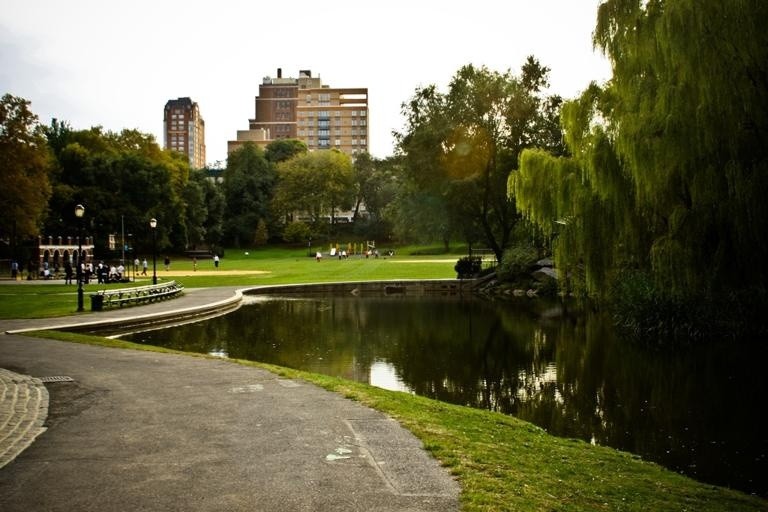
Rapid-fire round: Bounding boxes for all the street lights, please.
[75,203,85,312]
[149,217,157,284]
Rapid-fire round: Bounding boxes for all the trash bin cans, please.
[89,295,104,312]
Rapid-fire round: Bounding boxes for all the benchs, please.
[99,280,184,310]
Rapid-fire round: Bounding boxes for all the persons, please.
[213,253,219,267]
[164,255,170,271]
[10,260,19,280]
[134,255,139,275]
[140,255,148,275]
[41,257,125,286]
[314,244,384,263]
